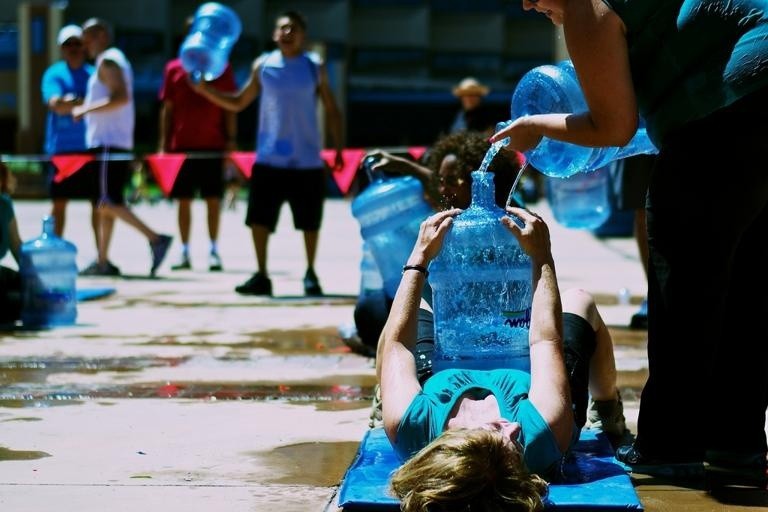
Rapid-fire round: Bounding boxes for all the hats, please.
[57,25,83,46]
[453,78,488,97]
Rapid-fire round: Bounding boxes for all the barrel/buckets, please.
[179,4,243,86]
[547,171,611,232]
[428,171,539,369]
[496,61,657,179]
[360,245,384,311]
[351,155,437,300]
[20,217,79,328]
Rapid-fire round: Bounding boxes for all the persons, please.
[620,155,648,329]
[157,15,238,271]
[449,78,492,133]
[0,162,49,337]
[373,204,633,511]
[189,8,346,300]
[70,15,174,276]
[41,23,97,274]
[356,130,521,209]
[492,0,768,491]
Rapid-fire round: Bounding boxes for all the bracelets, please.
[400,264,430,279]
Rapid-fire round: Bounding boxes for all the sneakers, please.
[585,387,625,438]
[80,260,118,276]
[616,445,647,465]
[150,236,173,272]
[338,322,376,357]
[172,254,221,270]
[304,277,321,295]
[236,272,271,294]
[370,384,382,427]
